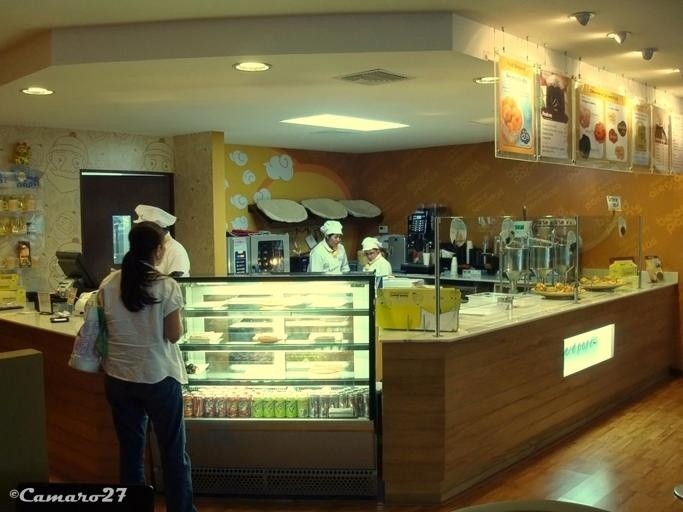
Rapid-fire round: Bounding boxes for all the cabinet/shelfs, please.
[0,171,44,271]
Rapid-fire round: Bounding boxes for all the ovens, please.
[226,230,290,274]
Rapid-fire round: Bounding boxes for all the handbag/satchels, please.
[69,305,102,374]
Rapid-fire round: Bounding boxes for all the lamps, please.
[569,11,656,61]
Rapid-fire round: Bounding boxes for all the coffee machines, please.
[405,205,450,271]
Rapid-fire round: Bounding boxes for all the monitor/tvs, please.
[55,250,99,290]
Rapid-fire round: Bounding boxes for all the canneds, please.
[183,387,369,419]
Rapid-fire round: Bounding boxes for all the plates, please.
[578,276,623,290]
[529,285,587,299]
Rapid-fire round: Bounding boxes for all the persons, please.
[133,204,192,278]
[96,220,199,512]
[362,236,393,276]
[306,220,351,273]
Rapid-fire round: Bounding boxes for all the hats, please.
[132,205,177,228]
[320,220,344,237]
[361,237,379,251]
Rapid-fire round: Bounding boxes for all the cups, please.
[450,257,458,276]
[422,253,430,266]
[499,96,524,145]
[466,240,474,265]
